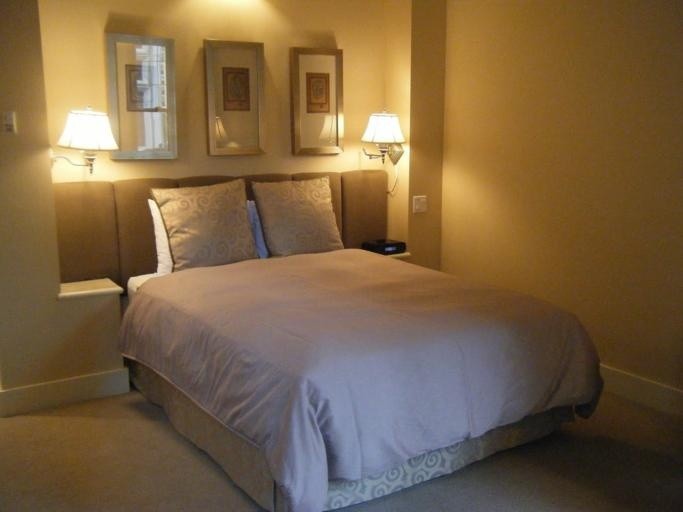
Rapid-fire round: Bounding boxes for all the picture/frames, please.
[106,31,178,161]
[203,39,267,156]
[289,45,345,156]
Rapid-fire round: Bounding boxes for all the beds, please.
[53,169,603,512]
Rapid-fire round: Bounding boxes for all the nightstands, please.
[389,251,413,259]
[58,277,131,404]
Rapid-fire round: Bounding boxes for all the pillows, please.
[146,176,345,273]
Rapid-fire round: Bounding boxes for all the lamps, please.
[361,110,406,165]
[50,107,119,174]
